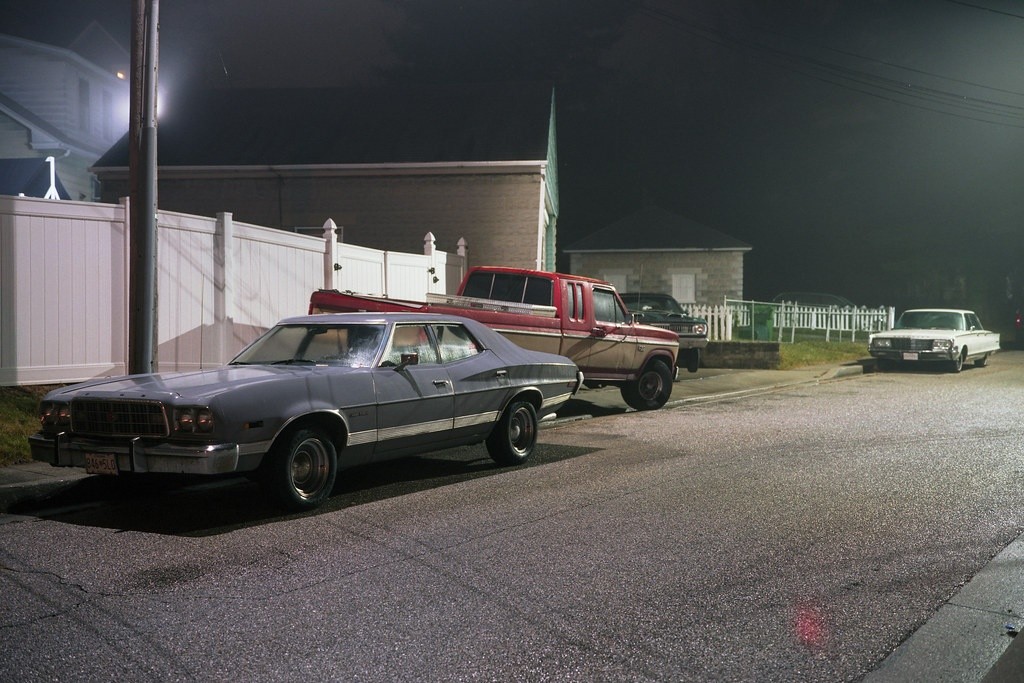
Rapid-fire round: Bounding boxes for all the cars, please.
[867,308,1000,374]
[619,292,709,373]
[28,311,586,515]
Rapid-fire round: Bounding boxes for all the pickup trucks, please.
[307,264,682,411]
